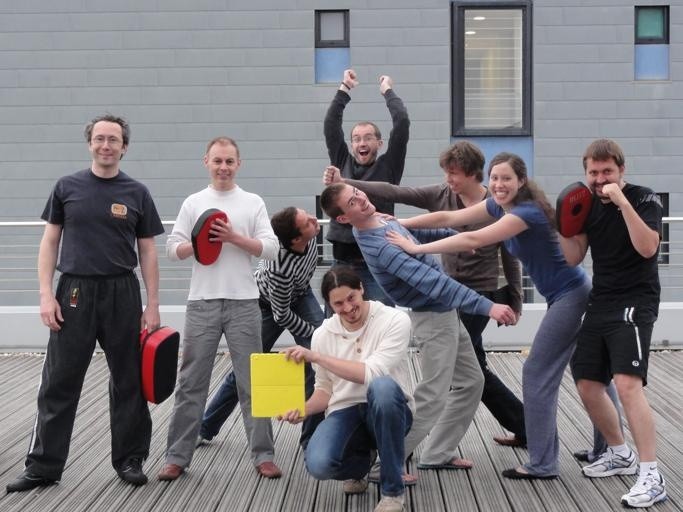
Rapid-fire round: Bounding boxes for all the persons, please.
[557,139,668,509]
[191,206,326,450]
[4,115,165,493]
[156,137,283,481]
[273,264,416,512]
[371,153,623,481]
[321,140,527,448]
[321,68,411,318]
[315,181,518,486]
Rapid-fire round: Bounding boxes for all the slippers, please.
[417,457,472,470]
[367,472,418,485]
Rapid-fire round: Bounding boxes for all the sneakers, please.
[502,467,557,480]
[582,448,637,477]
[343,473,368,493]
[373,493,405,512]
[574,448,595,463]
[117,460,148,485]
[620,473,667,507]
[6,470,48,492]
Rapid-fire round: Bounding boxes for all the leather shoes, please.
[256,462,282,478]
[492,434,518,446]
[158,462,181,479]
